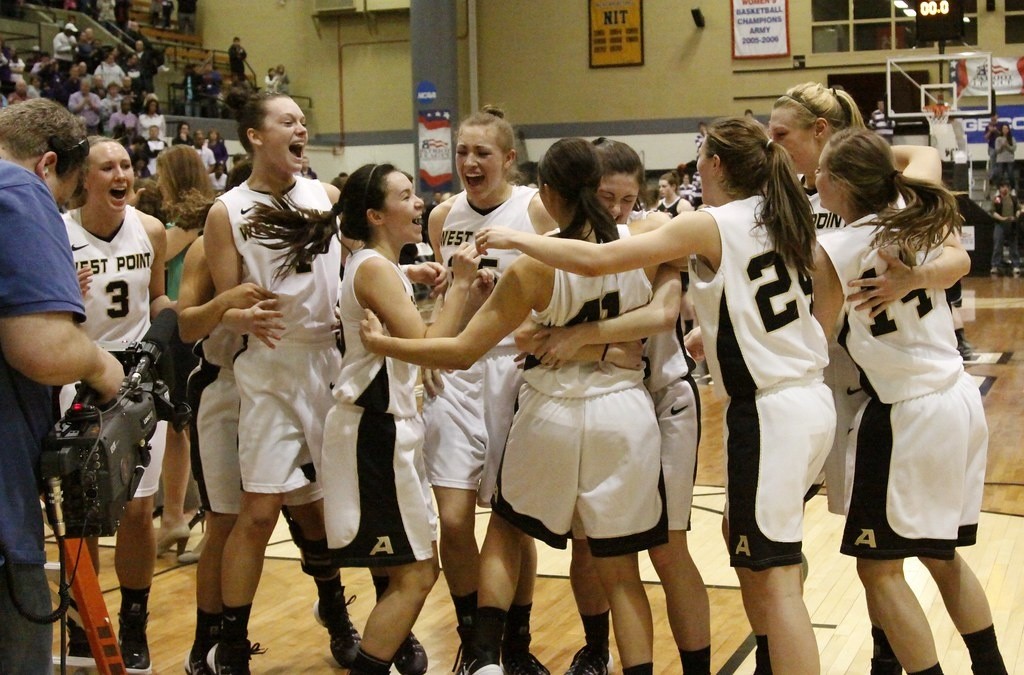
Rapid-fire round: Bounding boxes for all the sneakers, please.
[207,638,268,675]
[990,267,998,273]
[184,651,213,675]
[565,636,610,675]
[870,656,902,675]
[454,631,503,675]
[1012,267,1020,273]
[117,611,152,675]
[501,634,550,675]
[1010,188,1016,196]
[314,586,361,669]
[67,614,92,658]
[392,630,427,675]
[994,190,999,196]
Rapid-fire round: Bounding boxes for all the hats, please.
[66,23,77,32]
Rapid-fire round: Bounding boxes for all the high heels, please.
[155,525,190,556]
[152,505,163,523]
[187,506,206,533]
[177,551,201,562]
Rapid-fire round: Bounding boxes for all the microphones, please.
[130,307,179,390]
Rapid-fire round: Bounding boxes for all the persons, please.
[1,0,452,557]
[58,134,177,675]
[0,97,125,675]
[421,104,560,675]
[320,165,484,675]
[360,136,670,675]
[868,99,1024,278]
[204,92,428,675]
[632,109,802,382]
[682,83,972,675]
[474,118,838,675]
[178,155,364,674]
[810,128,1010,675]
[514,138,711,675]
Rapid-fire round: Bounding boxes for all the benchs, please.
[97,0,258,94]
